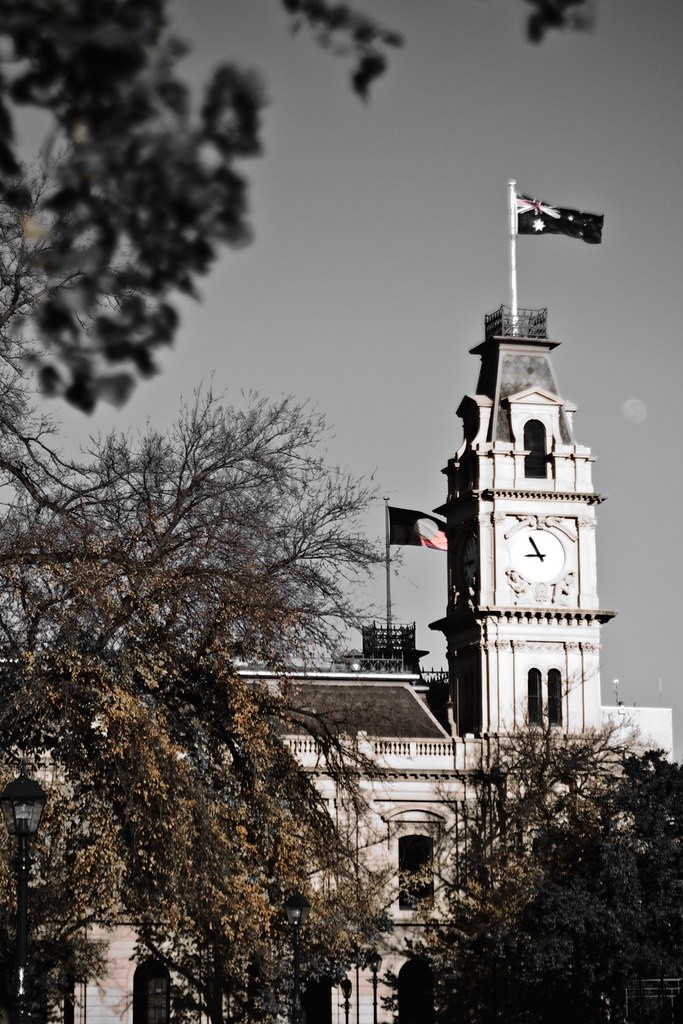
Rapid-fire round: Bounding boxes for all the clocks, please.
[513,529,565,584]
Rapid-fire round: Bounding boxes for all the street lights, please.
[0,760,49,1024]
[281,889,313,1024]
[339,974,352,1024]
[368,950,383,1024]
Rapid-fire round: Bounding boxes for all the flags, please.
[515,195,607,244]
[388,507,450,553]
